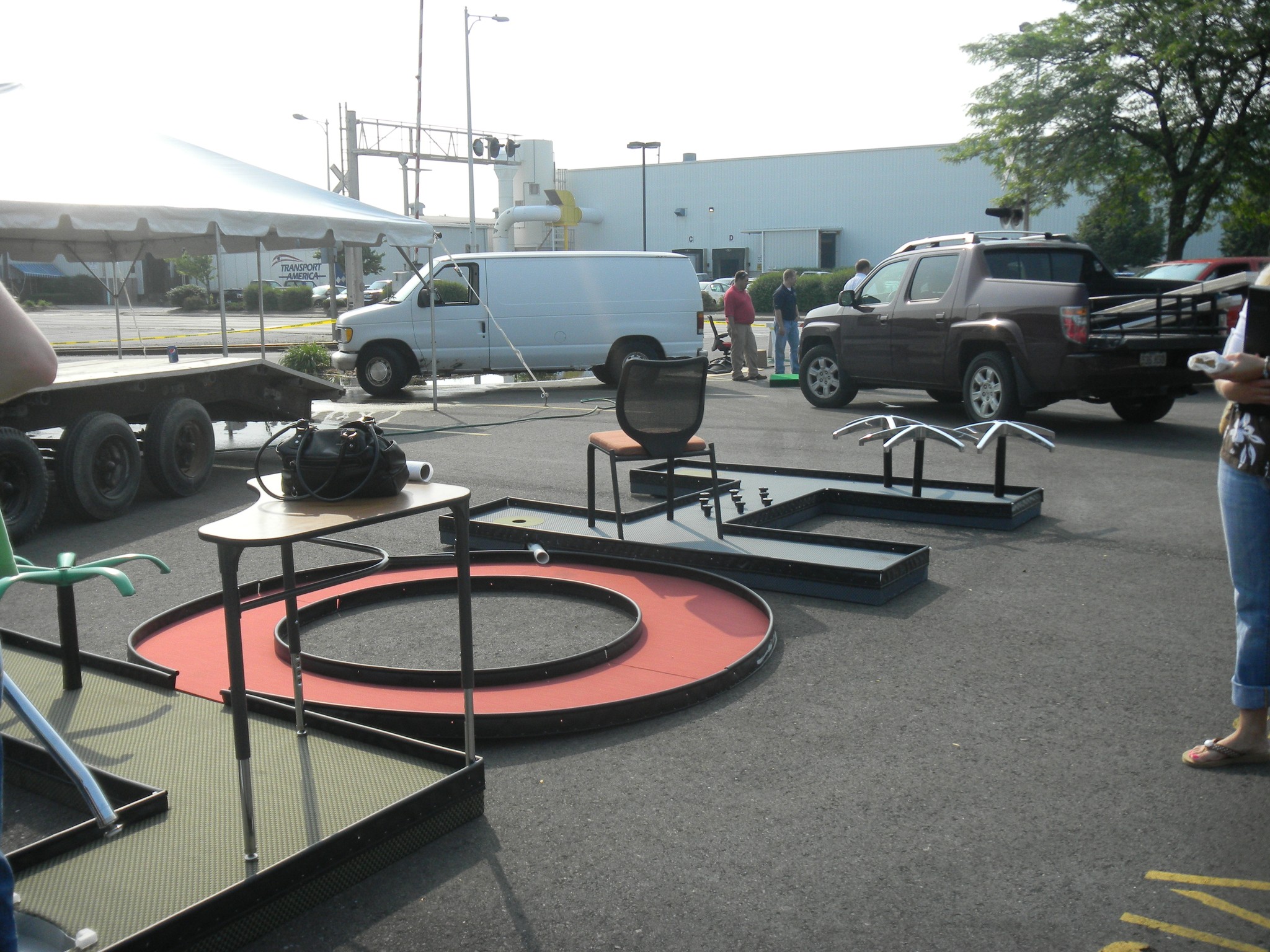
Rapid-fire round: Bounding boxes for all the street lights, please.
[626,141,661,251]
[292,113,331,193]
[463,6,510,254]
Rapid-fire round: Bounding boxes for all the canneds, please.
[167,345,179,363]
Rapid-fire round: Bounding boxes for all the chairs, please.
[587,356,724,541]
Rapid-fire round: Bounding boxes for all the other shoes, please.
[747,374,767,380]
[732,375,749,381]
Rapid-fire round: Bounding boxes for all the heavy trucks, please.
[0,274,348,550]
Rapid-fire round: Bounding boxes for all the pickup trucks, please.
[796,227,1231,432]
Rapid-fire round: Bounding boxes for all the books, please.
[1235,285,1270,407]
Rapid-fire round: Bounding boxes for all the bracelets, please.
[1263,356,1270,378]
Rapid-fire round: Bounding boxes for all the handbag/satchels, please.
[254,415,410,502]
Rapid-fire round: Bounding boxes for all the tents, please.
[0,80,438,411]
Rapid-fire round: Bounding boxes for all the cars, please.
[208,278,394,311]
[1110,255,1270,383]
[694,270,834,311]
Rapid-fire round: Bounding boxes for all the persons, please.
[723,270,767,381]
[1181,265,1270,769]
[844,259,877,297]
[773,269,800,375]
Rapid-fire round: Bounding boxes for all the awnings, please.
[10,263,64,278]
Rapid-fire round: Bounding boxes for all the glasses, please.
[741,272,747,277]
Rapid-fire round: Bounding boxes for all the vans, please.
[329,248,705,398]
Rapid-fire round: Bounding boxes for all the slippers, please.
[1182,738,1269,768]
